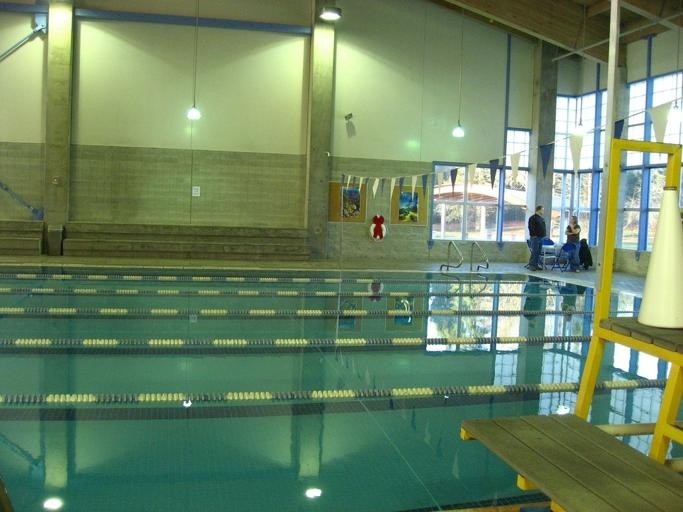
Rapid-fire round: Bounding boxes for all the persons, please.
[528,204,546,271]
[565,215,581,273]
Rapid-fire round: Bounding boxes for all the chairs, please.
[527,236,587,272]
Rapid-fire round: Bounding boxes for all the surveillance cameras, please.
[345,113,352,120]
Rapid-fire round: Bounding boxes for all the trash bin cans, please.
[46,223,64,256]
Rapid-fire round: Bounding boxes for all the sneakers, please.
[530,266,536,271]
[536,266,542,270]
